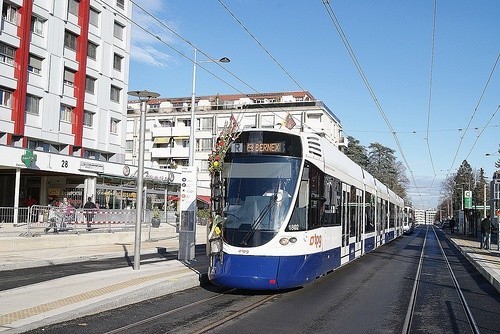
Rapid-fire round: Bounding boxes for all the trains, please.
[206,128,416,292]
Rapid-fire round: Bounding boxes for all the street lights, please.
[189,48,231,166]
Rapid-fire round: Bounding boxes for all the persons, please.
[449,217,455,234]
[83,197,97,231]
[44,208,57,232]
[57,198,69,230]
[480,218,490,250]
